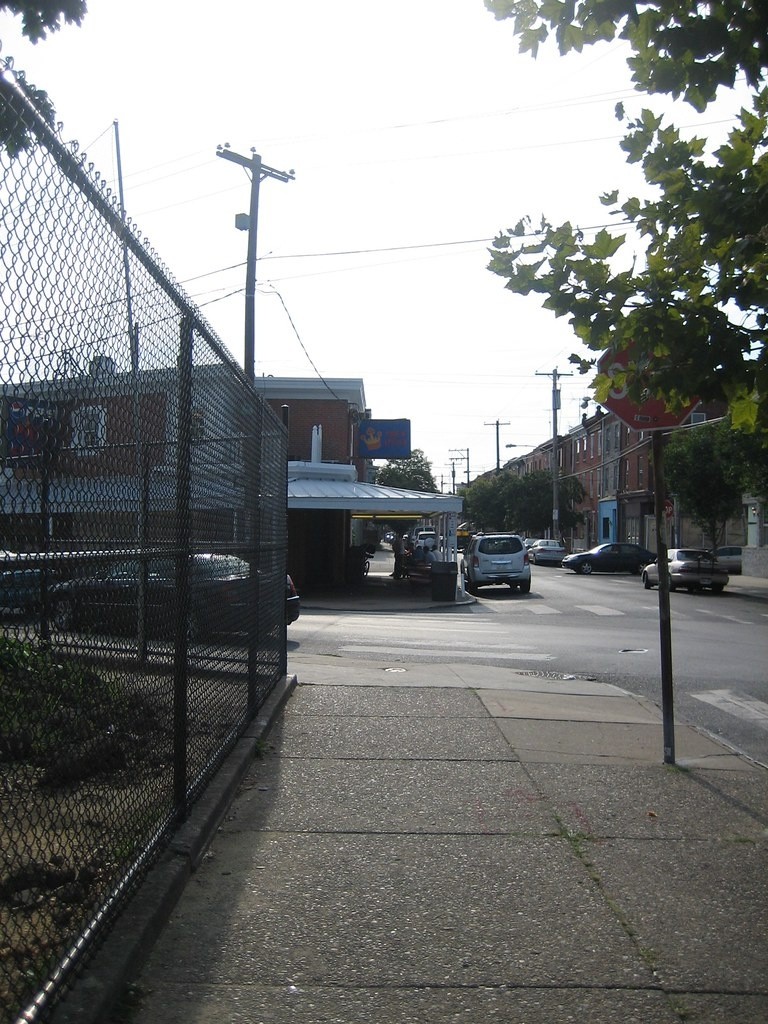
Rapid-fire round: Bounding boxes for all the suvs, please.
[460,531,532,595]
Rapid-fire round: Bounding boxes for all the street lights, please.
[505,443,561,545]
[463,469,502,532]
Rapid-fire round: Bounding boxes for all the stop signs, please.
[596,337,702,433]
[665,499,675,519]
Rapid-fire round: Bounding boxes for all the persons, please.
[389,530,443,580]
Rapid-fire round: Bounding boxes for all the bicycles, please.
[347,543,377,585]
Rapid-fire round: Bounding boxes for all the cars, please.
[0,542,47,616]
[384,521,479,551]
[44,550,301,645]
[522,536,567,565]
[641,546,730,594]
[560,541,658,577]
[700,544,743,573]
